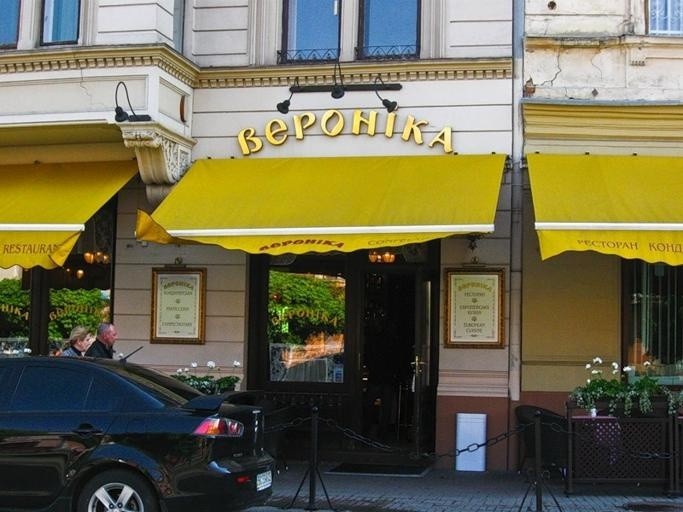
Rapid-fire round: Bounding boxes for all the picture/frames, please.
[150,264,206,347]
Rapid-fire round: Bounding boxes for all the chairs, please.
[516,404,570,493]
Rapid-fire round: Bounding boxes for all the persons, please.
[83,323,119,359]
[52,348,61,357]
[58,326,91,358]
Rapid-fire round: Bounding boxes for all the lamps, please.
[276,75,300,114]
[367,249,395,264]
[111,81,151,122]
[331,60,344,100]
[374,76,400,114]
[82,245,111,266]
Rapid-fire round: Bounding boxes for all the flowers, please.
[568,356,676,415]
[174,358,242,393]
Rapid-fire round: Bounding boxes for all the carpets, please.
[322,461,433,479]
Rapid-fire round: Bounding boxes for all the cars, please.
[1,354,278,512]
